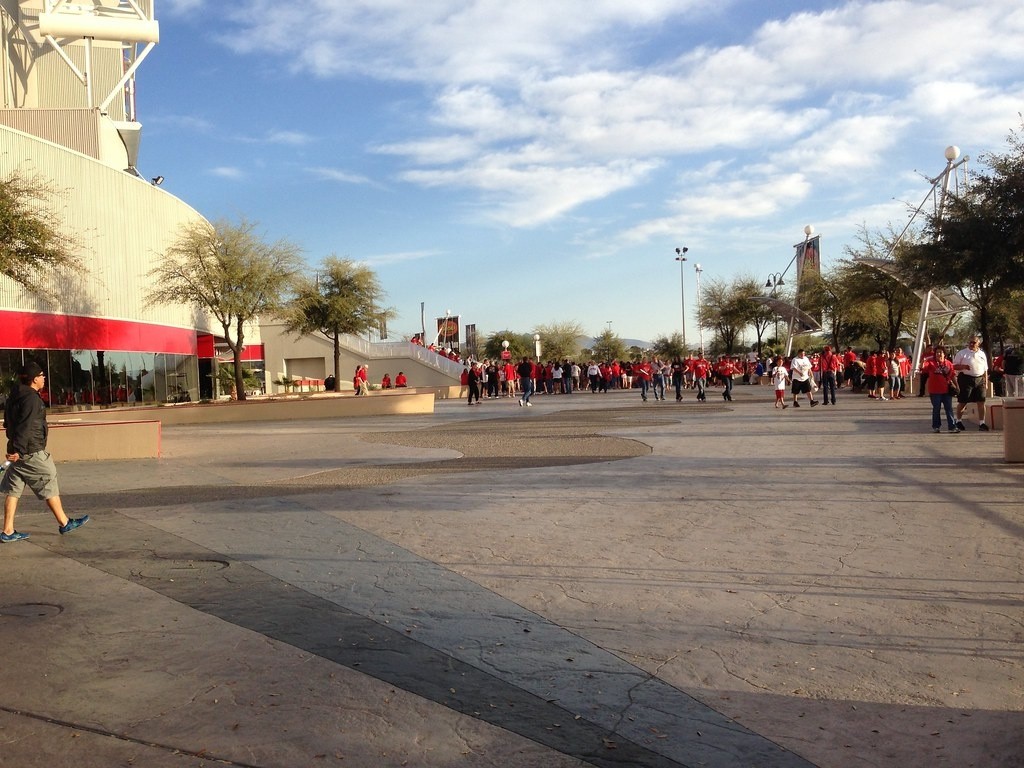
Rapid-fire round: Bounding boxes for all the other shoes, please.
[832,403,835,404]
[899,393,905,397]
[822,402,829,405]
[894,396,901,399]
[880,396,888,400]
[876,397,879,400]
[868,394,877,398]
[979,423,989,432]
[948,427,960,433]
[932,428,940,433]
[890,397,895,400]
[917,394,924,397]
[468,382,819,410]
[955,421,965,430]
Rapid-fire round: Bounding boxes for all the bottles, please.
[0,460,11,473]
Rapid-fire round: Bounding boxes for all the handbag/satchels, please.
[988,370,1003,382]
[948,382,959,397]
[810,379,818,393]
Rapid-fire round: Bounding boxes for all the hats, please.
[726,355,731,358]
[775,355,782,361]
[824,346,831,351]
[18,362,42,384]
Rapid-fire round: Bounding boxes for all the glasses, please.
[36,373,44,377]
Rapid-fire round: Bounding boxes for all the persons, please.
[394,372,407,388]
[382,374,393,389]
[924,346,961,433]
[411,336,423,346]
[440,347,460,362]
[992,349,1012,396]
[39,385,142,406]
[428,343,434,351]
[354,364,370,395]
[953,334,989,430]
[0,362,89,542]
[919,345,953,396]
[459,332,912,409]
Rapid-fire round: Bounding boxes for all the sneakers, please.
[59,514,90,535]
[0,530,30,542]
